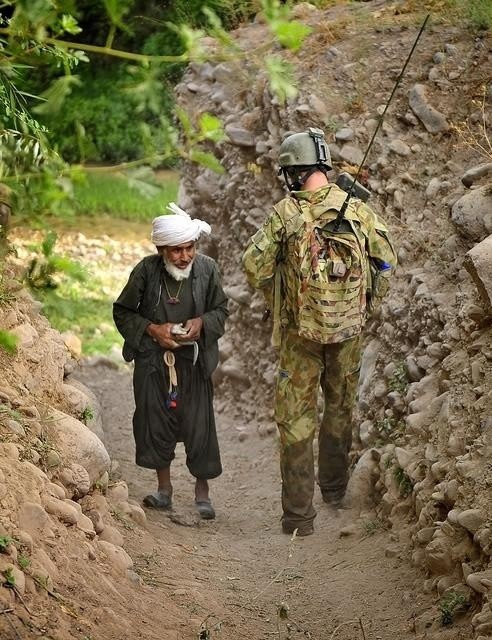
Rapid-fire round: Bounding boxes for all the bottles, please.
[378,263,390,277]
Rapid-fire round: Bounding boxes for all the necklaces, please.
[162,272,185,305]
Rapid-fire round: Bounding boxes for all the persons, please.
[242,131,398,538]
[111,202,232,521]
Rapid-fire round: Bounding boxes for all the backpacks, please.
[275,193,363,345]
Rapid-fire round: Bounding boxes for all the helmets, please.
[277,132,332,171]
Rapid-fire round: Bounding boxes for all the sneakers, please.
[144,492,171,509]
[194,498,215,519]
[322,492,343,504]
[281,519,315,535]
